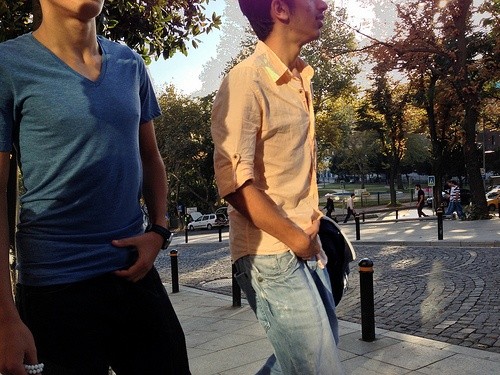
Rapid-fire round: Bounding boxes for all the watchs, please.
[145,223,174,249]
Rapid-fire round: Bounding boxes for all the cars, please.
[469,186,500,211]
[426,187,470,208]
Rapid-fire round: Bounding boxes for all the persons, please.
[344,193,359,224]
[0,0,192,375]
[415,184,430,217]
[320,197,339,224]
[445,180,464,217]
[211,0,343,375]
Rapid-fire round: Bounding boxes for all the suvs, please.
[188,212,227,230]
